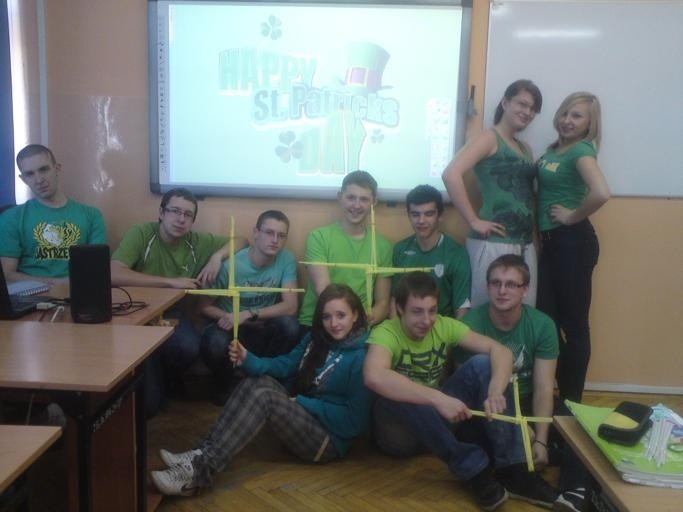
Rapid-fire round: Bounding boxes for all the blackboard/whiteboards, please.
[482,0,683,198]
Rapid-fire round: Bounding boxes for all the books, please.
[563,399,682,489]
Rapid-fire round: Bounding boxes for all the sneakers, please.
[149,460,201,497]
[465,473,510,511]
[494,467,563,509]
[159,449,203,468]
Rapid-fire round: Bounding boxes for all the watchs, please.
[247,306,258,321]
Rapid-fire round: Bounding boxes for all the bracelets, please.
[533,439,551,450]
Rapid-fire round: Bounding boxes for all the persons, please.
[151,284,377,497]
[0,144,107,285]
[441,79,537,311]
[390,182,471,323]
[297,170,393,345]
[452,251,602,512]
[361,267,568,511]
[110,186,249,419]
[535,91,610,427]
[195,209,302,407]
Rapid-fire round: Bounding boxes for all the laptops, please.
[0,259,54,321]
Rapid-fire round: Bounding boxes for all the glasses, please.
[163,207,195,220]
[511,99,537,116]
[259,229,286,242]
[488,278,525,290]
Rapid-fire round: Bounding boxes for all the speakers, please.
[69,244,112,324]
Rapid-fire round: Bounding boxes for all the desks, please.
[554,415,683,512]
[1,320,176,511]
[0,426,62,493]
[12,285,186,324]
